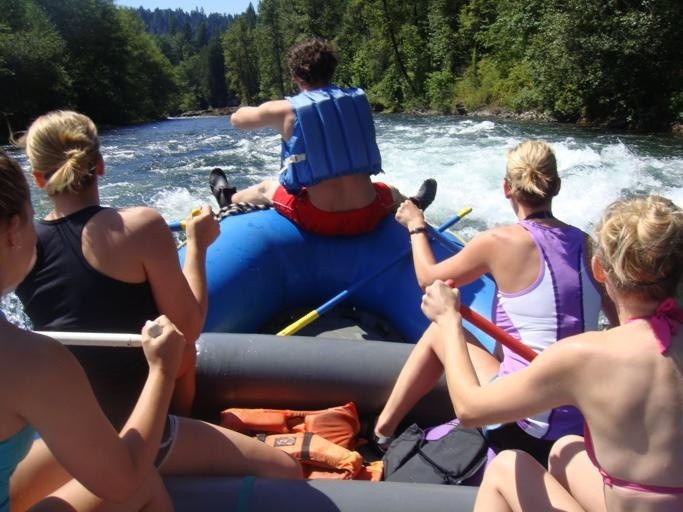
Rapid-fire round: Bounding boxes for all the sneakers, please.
[209,167,236,209]
[410,178,438,210]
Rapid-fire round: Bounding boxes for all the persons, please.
[10,108,303,477]
[421,195,683,512]
[209,40,437,235]
[0,156,187,511]
[373,139,621,486]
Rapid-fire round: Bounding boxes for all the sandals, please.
[361,409,396,457]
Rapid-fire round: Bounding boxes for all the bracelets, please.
[409,227,428,235]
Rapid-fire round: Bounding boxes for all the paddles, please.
[276,208,472,336]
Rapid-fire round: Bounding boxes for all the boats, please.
[113,192,570,512]
